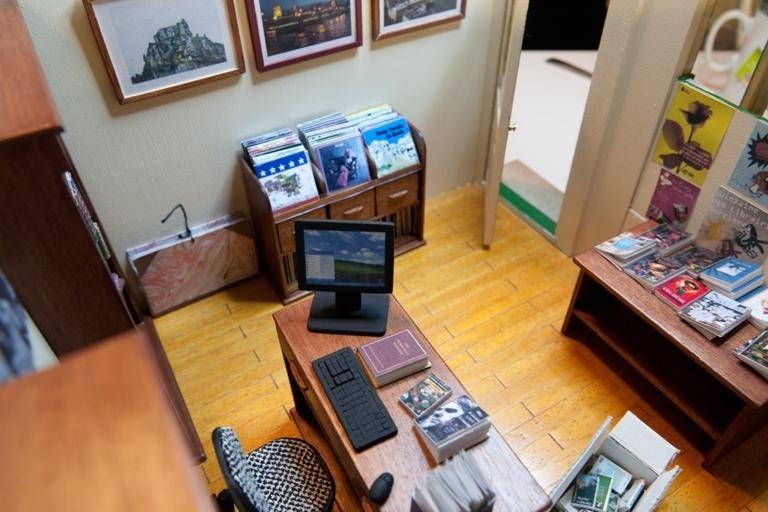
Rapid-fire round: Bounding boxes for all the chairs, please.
[209,425,337,512]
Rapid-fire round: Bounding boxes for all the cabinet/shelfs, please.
[234,109,428,308]
[0,2,144,374]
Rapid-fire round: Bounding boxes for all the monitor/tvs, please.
[295,219,395,337]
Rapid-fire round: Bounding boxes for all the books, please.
[593,218,768,377]
[240,100,421,287]
[399,370,454,419]
[410,393,647,512]
[64,170,121,297]
[355,327,433,390]
[645,80,767,267]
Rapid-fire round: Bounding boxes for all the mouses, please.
[369,473,393,506]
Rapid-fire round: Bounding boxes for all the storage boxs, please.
[544,408,688,512]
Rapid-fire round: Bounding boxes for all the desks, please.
[270,283,558,511]
[557,215,767,471]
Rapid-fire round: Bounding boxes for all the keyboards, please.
[311,346,398,452]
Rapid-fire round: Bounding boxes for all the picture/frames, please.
[82,1,246,106]
[371,1,466,42]
[246,1,363,73]
